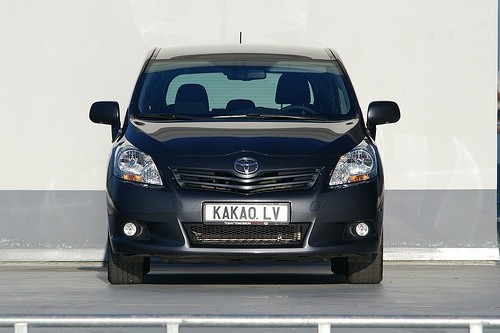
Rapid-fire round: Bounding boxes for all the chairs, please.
[225,100,256,111]
[275,74,311,111]
[175,84,210,114]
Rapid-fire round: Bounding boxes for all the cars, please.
[88,42,401,285]
